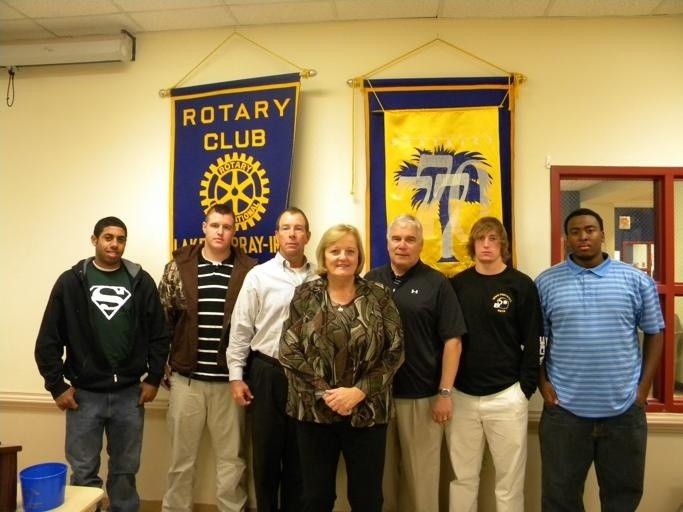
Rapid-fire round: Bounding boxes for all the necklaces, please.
[329,299,345,312]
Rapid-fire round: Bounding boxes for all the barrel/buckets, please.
[19,462,68,512]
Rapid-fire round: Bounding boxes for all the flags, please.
[359,76,518,282]
[169,72,301,263]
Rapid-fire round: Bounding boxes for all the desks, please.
[15,486,105,512]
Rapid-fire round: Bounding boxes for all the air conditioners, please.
[0,33,133,68]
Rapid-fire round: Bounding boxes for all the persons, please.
[156,204,260,512]
[534,206,667,511]
[361,213,469,512]
[443,218,544,511]
[276,224,405,511]
[33,215,169,511]
[223,205,324,511]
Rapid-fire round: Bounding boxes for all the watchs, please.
[436,386,452,398]
